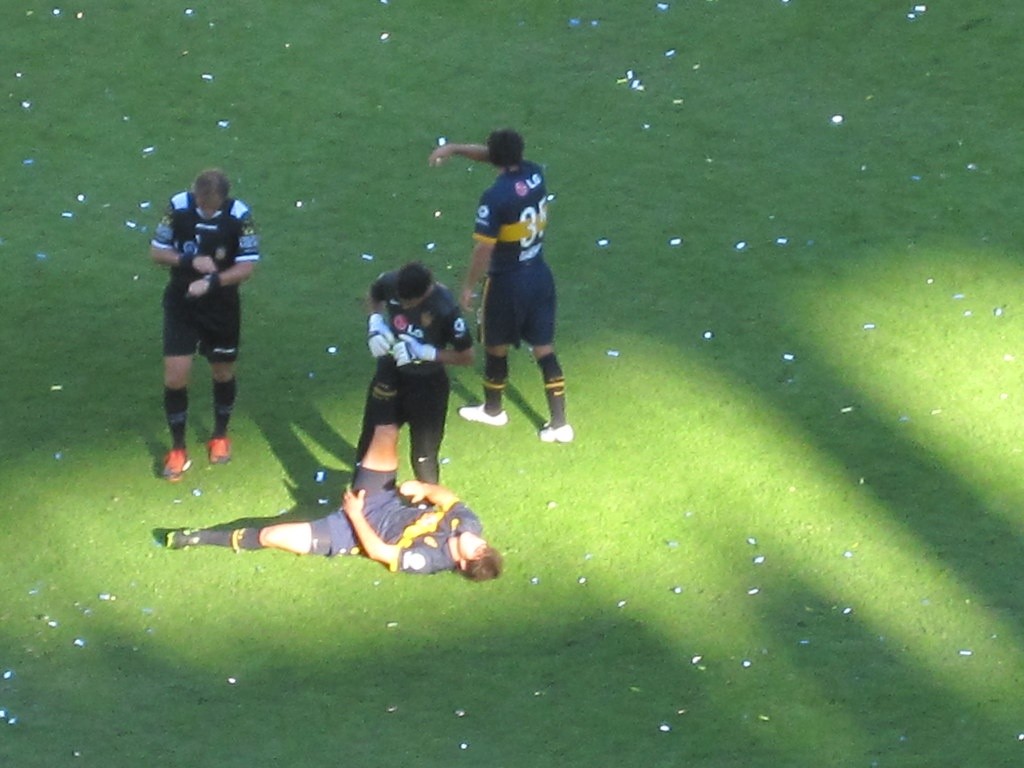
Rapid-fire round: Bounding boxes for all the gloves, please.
[367,313,394,358]
[392,333,437,367]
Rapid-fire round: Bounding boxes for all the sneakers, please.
[459,403,509,426]
[162,449,188,483]
[539,421,573,443]
[166,528,197,551]
[209,437,230,465]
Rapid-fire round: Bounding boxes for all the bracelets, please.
[206,272,220,292]
[180,253,195,270]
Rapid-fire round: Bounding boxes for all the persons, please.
[147,168,260,484]
[428,128,575,447]
[166,347,504,586]
[349,263,476,486]
